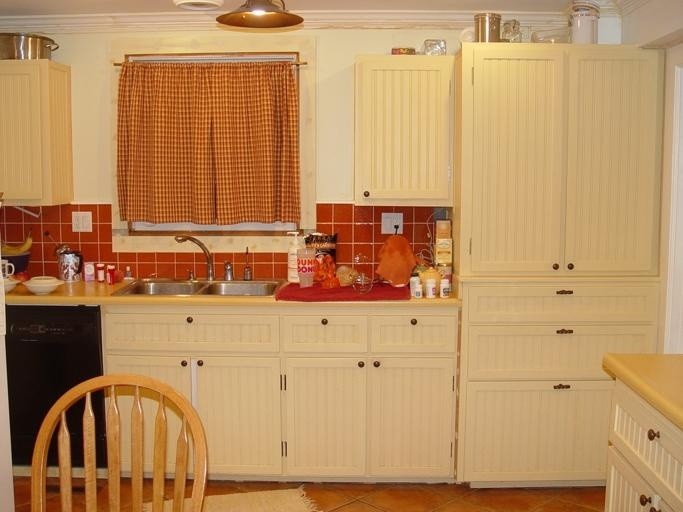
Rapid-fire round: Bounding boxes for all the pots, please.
[0,32,58,60]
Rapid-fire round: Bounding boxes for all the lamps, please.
[216,0,303,27]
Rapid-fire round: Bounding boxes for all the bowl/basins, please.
[1,252,31,275]
[5,284,15,293]
[24,284,58,295]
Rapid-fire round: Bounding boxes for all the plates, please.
[5,279,20,284]
[22,277,63,284]
[276,279,410,301]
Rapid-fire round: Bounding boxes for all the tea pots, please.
[417,266,446,294]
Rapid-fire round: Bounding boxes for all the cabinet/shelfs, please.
[600,351,683,512]
[0,59,73,205]
[350,44,671,489]
[3,278,462,487]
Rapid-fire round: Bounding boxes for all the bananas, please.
[1,231,32,255]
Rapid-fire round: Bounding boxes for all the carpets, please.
[143,485,318,512]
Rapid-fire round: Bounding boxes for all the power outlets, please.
[71,211,93,232]
[381,212,403,235]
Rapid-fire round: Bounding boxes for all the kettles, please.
[55,247,84,282]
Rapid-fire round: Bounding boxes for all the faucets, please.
[173,235,214,281]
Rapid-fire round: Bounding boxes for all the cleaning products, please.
[286,231,304,285]
[287,230,306,282]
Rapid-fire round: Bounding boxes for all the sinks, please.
[199,281,280,298]
[109,278,204,296]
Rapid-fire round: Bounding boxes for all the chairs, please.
[31,374,207,512]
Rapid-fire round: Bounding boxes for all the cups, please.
[351,255,374,293]
[297,248,316,288]
[1,260,14,278]
